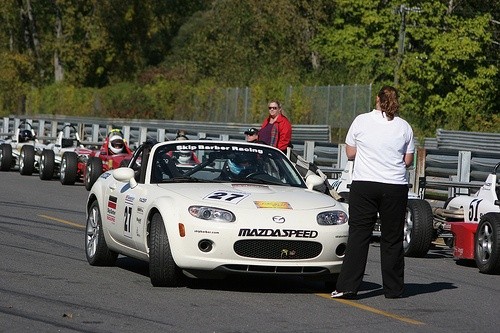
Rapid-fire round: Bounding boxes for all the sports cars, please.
[85,140,351,288]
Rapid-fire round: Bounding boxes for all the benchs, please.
[162,168,226,181]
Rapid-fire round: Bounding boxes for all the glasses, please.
[268,107,277,110]
[250,133,254,136]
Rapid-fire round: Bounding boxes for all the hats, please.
[244,128,259,134]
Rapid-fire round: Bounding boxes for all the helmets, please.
[227,156,258,176]
[176,147,192,162]
[108,136,125,154]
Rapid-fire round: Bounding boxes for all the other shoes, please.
[331,289,353,298]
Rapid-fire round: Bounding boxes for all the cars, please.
[0,121,142,191]
[328,159,433,258]
[440,163,500,273]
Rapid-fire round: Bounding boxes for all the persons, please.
[108,135,126,156]
[213,145,258,182]
[244,128,266,144]
[258,100,292,180]
[174,151,192,164]
[330,86,414,298]
